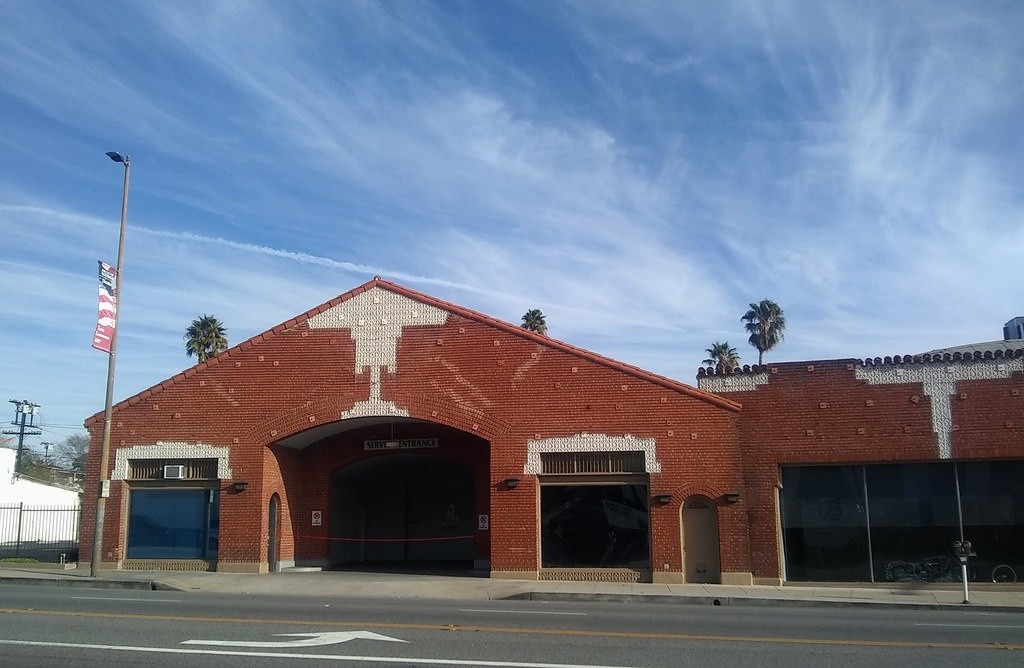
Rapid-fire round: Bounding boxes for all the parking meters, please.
[954,541,971,604]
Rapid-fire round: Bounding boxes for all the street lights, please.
[92,151,131,577]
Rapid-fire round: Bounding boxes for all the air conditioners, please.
[164,465,184,479]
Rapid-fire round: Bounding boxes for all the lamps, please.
[724,493,740,503]
[234,480,249,491]
[505,478,520,488]
[386,423,399,448]
[657,493,673,504]
